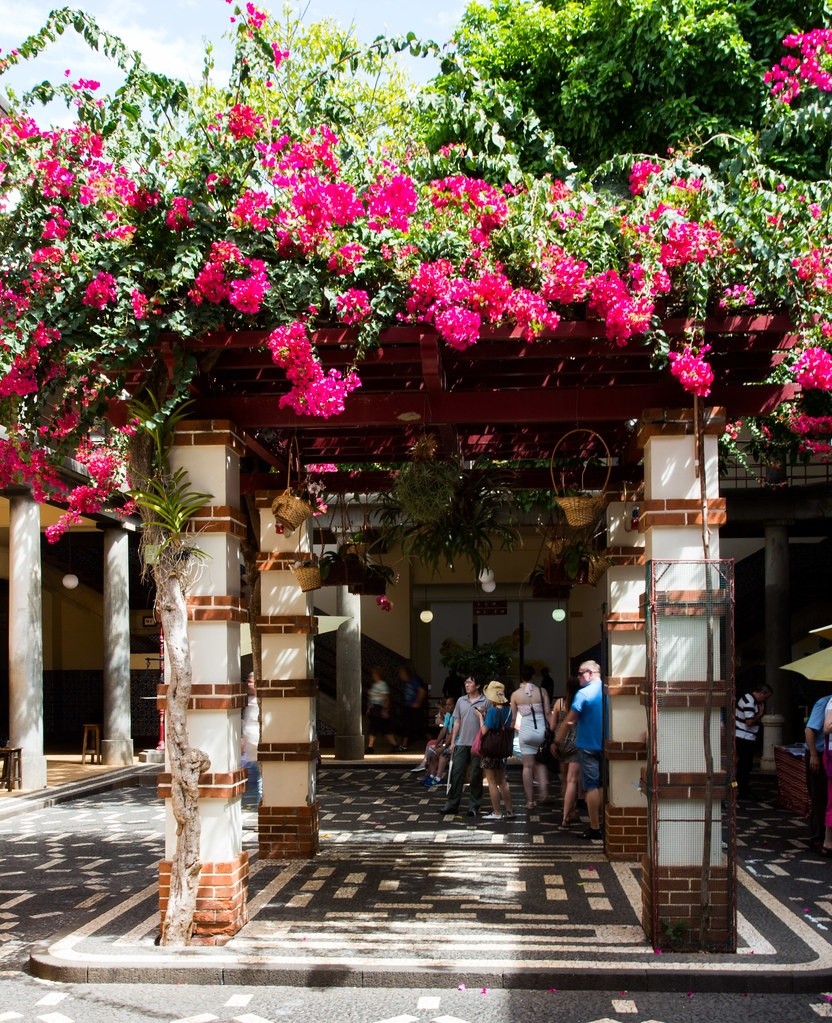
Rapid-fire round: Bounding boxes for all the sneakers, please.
[426,778,444,787]
[503,810,515,816]
[420,774,434,783]
[481,812,503,819]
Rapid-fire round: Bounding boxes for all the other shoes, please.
[364,747,374,754]
[411,766,427,772]
[467,804,480,817]
[559,821,570,830]
[438,807,459,814]
[581,826,603,840]
[390,744,407,753]
[417,774,431,780]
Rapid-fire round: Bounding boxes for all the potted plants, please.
[305,544,363,586]
[544,540,599,585]
[528,563,573,600]
[348,564,394,596]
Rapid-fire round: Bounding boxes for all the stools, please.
[82,724,103,764]
[0,746,22,791]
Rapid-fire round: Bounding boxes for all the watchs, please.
[443,743,447,748]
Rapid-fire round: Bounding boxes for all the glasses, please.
[579,669,589,676]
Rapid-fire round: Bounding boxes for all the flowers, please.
[0,0,832,613]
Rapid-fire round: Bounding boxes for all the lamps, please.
[479,543,494,583]
[481,581,496,593]
[553,601,565,623]
[61,527,79,589]
[420,586,433,624]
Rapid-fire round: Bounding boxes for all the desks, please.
[773,743,813,817]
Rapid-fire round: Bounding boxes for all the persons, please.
[441,669,467,706]
[365,666,398,753]
[474,680,515,819]
[803,695,832,857]
[550,659,609,839]
[436,670,489,815]
[510,659,552,810]
[540,666,553,703]
[735,683,774,800]
[411,696,459,786]
[241,672,262,804]
[399,664,427,751]
[550,675,581,827]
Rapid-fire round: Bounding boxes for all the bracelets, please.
[553,741,560,747]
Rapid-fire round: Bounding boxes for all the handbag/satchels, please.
[470,727,485,755]
[482,707,515,756]
[539,728,557,766]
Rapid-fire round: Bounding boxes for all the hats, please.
[483,680,508,703]
